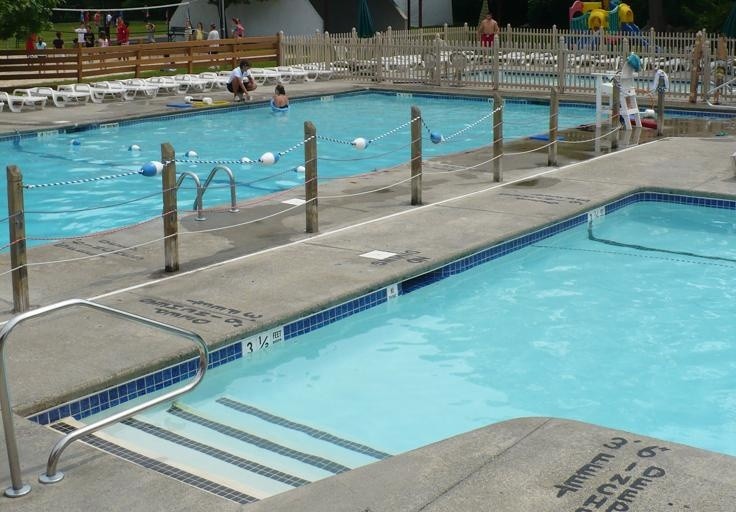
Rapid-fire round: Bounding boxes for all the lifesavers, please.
[271,98,290,112]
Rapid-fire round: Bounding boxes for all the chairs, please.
[248,63,346,87]
[363,47,719,77]
[0,82,91,114]
[166,70,230,94]
[91,75,181,104]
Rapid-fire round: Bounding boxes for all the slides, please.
[574,31,599,56]
[624,22,661,53]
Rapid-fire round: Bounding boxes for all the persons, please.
[23,10,245,75]
[225,58,259,102]
[479,11,501,48]
[270,82,290,118]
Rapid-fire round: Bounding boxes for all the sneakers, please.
[208,66,221,70]
[242,94,252,101]
[234,95,241,102]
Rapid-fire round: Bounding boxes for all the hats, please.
[240,60,250,69]
